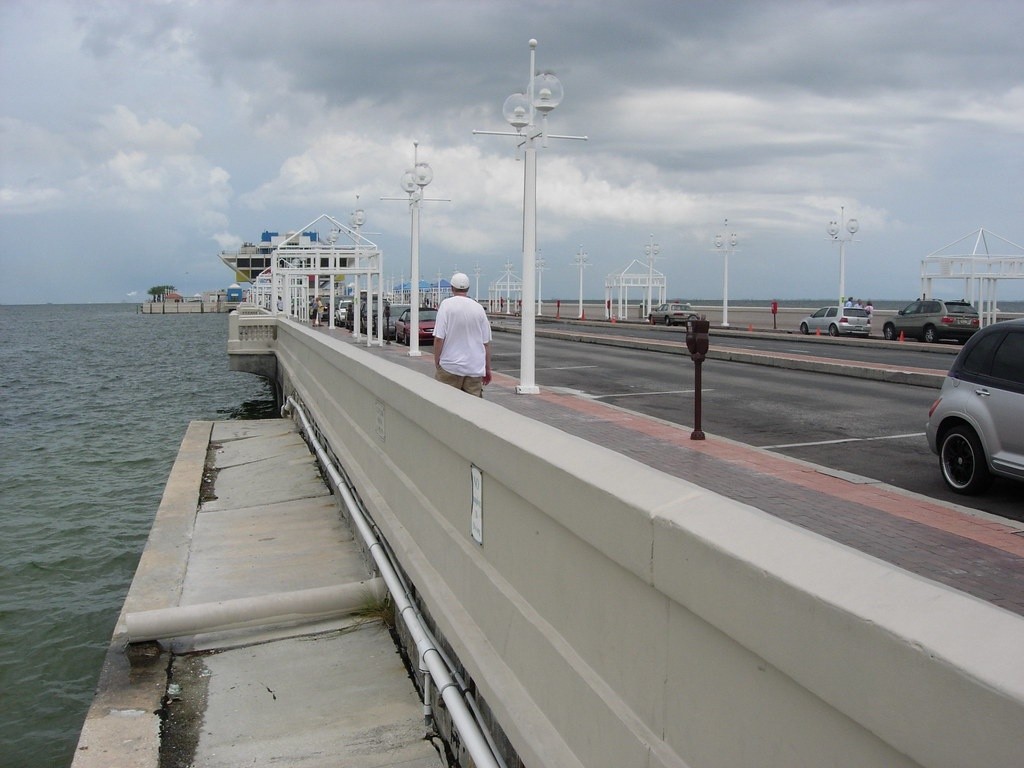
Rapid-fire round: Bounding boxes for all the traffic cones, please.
[748,323,752,332]
[611,313,616,324]
[900,330,905,342]
[816,326,820,336]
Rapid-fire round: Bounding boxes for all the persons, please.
[433,273,492,398]
[846,297,873,334]
[312,297,324,326]
[277,296,283,311]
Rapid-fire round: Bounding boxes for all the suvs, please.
[882,295,981,345]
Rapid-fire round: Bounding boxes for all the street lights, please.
[575,243,588,319]
[714,216,737,327]
[644,233,659,321]
[460,0,588,394]
[824,204,861,307]
[498,256,518,315]
[326,192,382,344]
[465,263,488,303]
[534,248,551,316]
[379,139,451,358]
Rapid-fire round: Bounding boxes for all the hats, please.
[450,273,470,289]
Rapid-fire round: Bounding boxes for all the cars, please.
[648,302,700,326]
[259,291,438,346]
[799,306,871,338]
[926,319,1023,494]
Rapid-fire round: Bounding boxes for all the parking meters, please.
[686,313,709,440]
